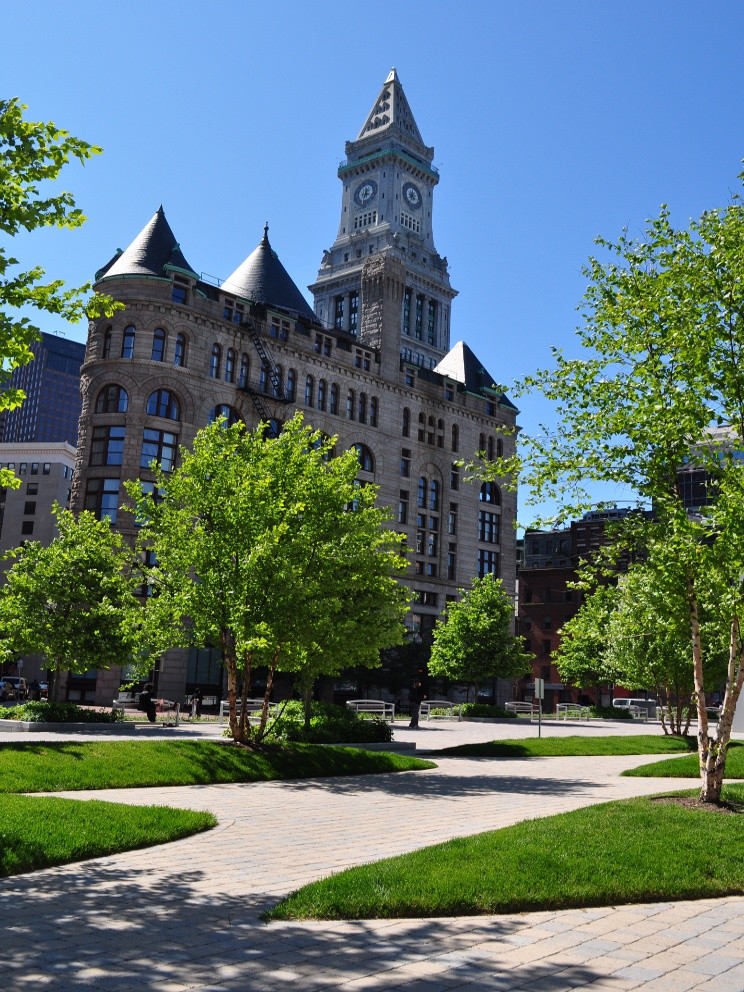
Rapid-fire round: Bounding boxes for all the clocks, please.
[353,179,378,208]
[401,181,423,211]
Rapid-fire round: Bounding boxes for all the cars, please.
[0,676,48,703]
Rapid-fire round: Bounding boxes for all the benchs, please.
[419,700,462,723]
[346,700,395,723]
[219,700,278,725]
[504,701,542,720]
[185,694,216,715]
[556,703,589,722]
[112,698,181,727]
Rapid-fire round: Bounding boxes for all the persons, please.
[28,679,39,700]
[137,683,156,723]
[408,679,424,729]
[189,688,204,718]
